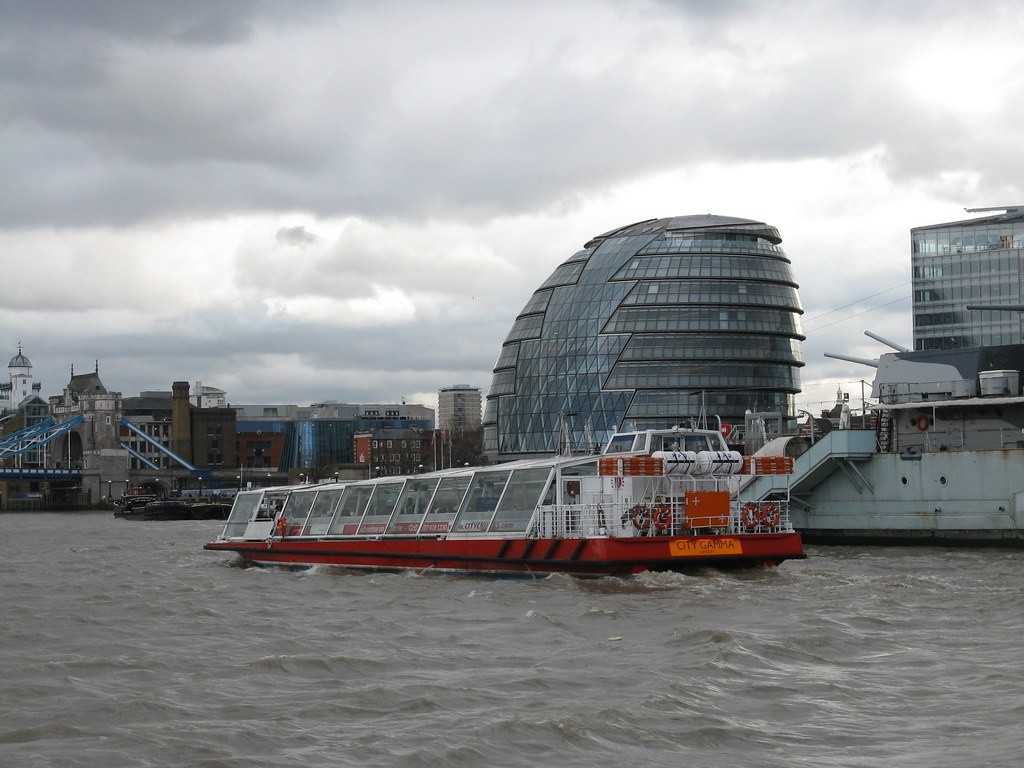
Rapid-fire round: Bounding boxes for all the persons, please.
[292,489,463,518]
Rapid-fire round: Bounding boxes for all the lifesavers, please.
[762,504,779,527]
[633,507,650,530]
[915,414,929,431]
[742,505,760,528]
[653,507,671,529]
[276,518,287,536]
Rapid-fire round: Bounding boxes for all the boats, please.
[109,494,276,520]
[203,424,809,582]
